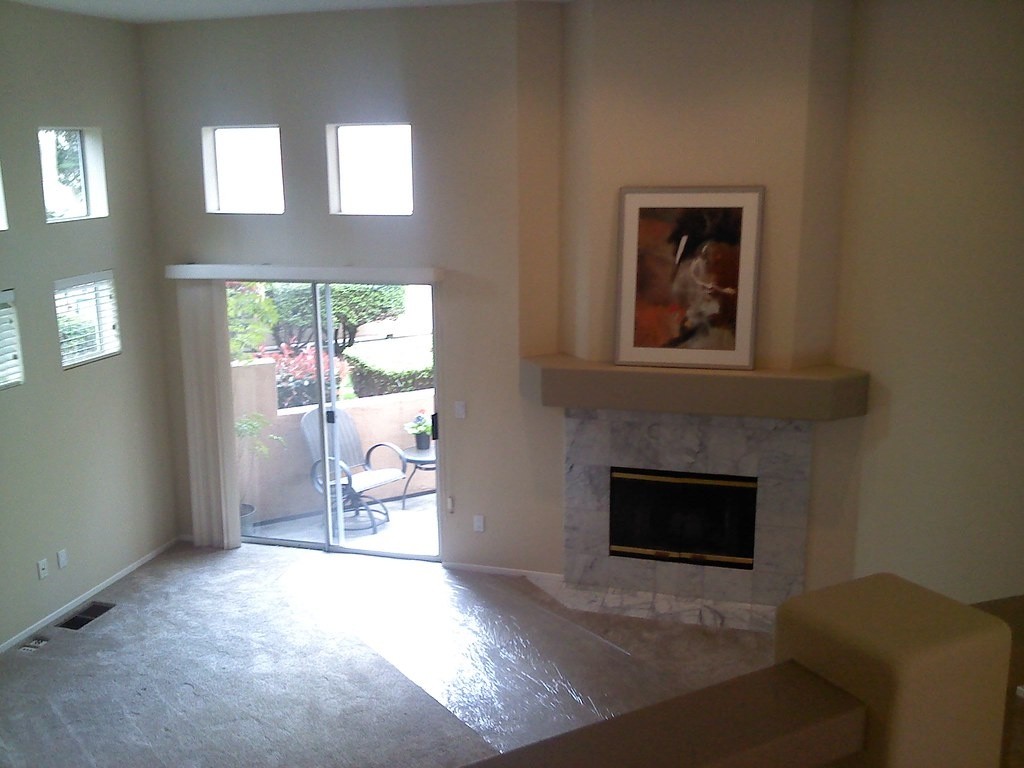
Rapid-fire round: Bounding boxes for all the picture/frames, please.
[613,185,766,373]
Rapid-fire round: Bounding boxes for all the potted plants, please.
[234,412,288,536]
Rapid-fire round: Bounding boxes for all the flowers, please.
[404,409,432,436]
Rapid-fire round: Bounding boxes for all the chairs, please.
[299,406,406,535]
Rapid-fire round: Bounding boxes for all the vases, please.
[415,434,431,450]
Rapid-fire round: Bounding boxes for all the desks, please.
[401,445,437,510]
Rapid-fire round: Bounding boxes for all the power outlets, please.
[37,559,49,579]
[57,549,69,569]
[472,515,485,533]
